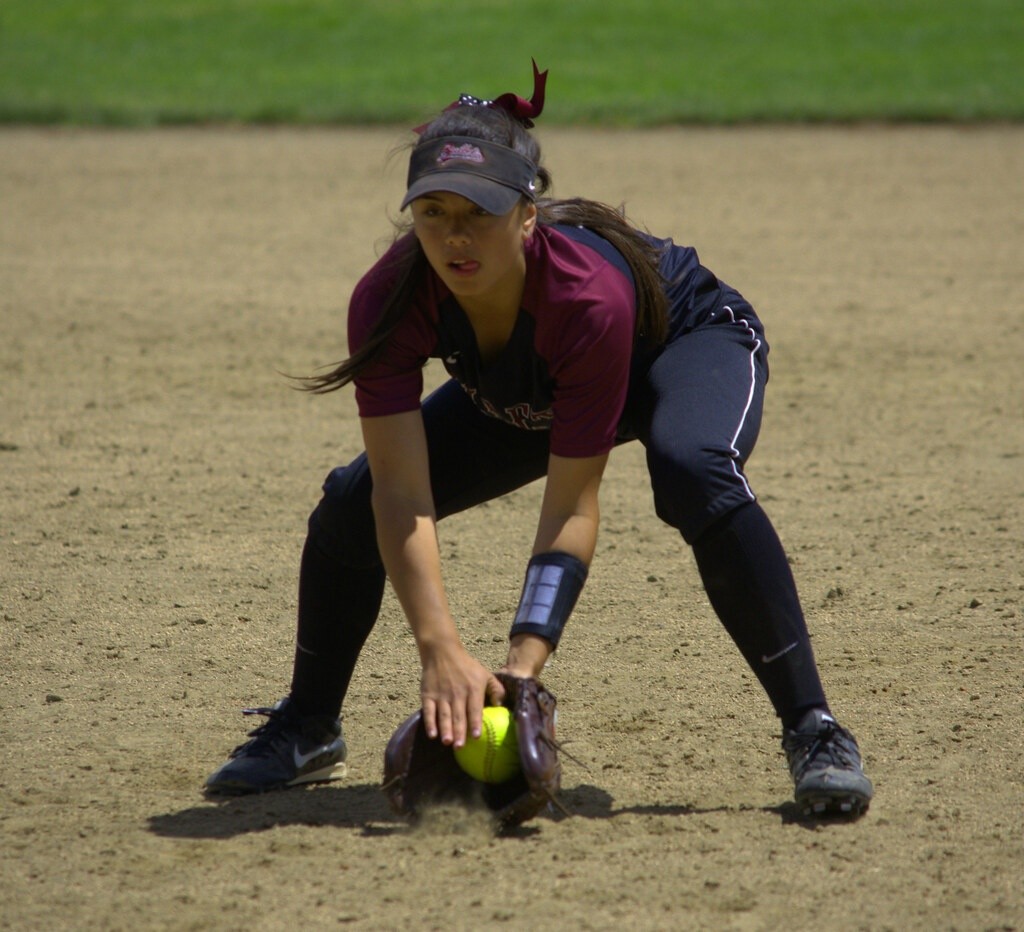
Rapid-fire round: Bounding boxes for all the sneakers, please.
[201,698,347,795]
[782,707,872,819]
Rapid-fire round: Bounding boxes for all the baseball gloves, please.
[380,673,591,828]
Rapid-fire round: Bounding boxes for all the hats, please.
[398,134,537,217]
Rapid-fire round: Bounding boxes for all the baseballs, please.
[453,705,521,783]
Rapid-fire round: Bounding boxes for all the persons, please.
[201,60,873,819]
